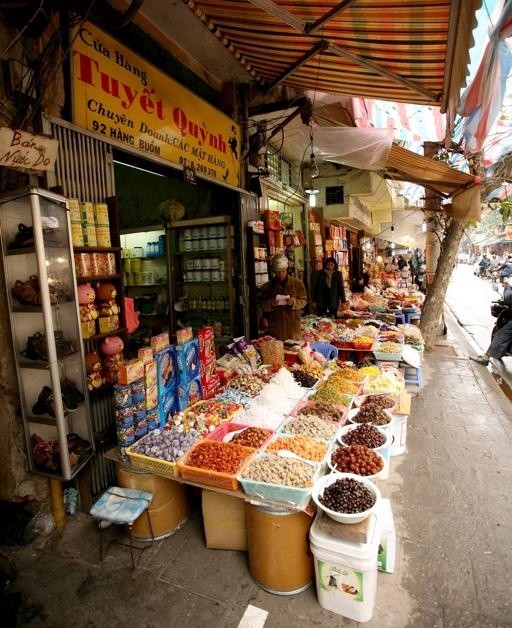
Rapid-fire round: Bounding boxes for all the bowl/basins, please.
[353,393,397,413]
[310,472,381,524]
[326,446,386,477]
[336,423,392,449]
[347,408,394,426]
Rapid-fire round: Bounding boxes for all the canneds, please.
[182,225,234,282]
[122,259,141,287]
[123,248,130,258]
[68,198,112,247]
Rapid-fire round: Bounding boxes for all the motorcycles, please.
[491,300,512,356]
[479,263,512,288]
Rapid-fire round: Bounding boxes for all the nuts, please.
[352,321,403,353]
[130,362,404,513]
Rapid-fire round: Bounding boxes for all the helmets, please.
[271,253,289,272]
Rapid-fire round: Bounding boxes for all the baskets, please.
[126,381,362,507]
[332,335,403,362]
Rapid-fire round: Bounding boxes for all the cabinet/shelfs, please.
[1,186,129,479]
[118,214,236,345]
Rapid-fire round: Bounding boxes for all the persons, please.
[351,276,365,293]
[397,254,407,269]
[312,256,347,318]
[415,264,427,294]
[477,248,512,283]
[469,277,512,366]
[255,254,309,342]
[408,260,415,284]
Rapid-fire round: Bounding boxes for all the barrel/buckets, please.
[113,465,191,543]
[308,509,379,623]
[245,503,312,595]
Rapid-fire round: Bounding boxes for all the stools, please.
[89,487,156,569]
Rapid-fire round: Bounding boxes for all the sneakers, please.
[11,271,86,415]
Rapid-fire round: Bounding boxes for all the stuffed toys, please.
[78,282,99,322]
[95,280,121,316]
[101,335,125,372]
[85,349,106,392]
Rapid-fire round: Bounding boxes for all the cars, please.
[474,255,502,276]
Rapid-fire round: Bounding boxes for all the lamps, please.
[304,122,320,209]
